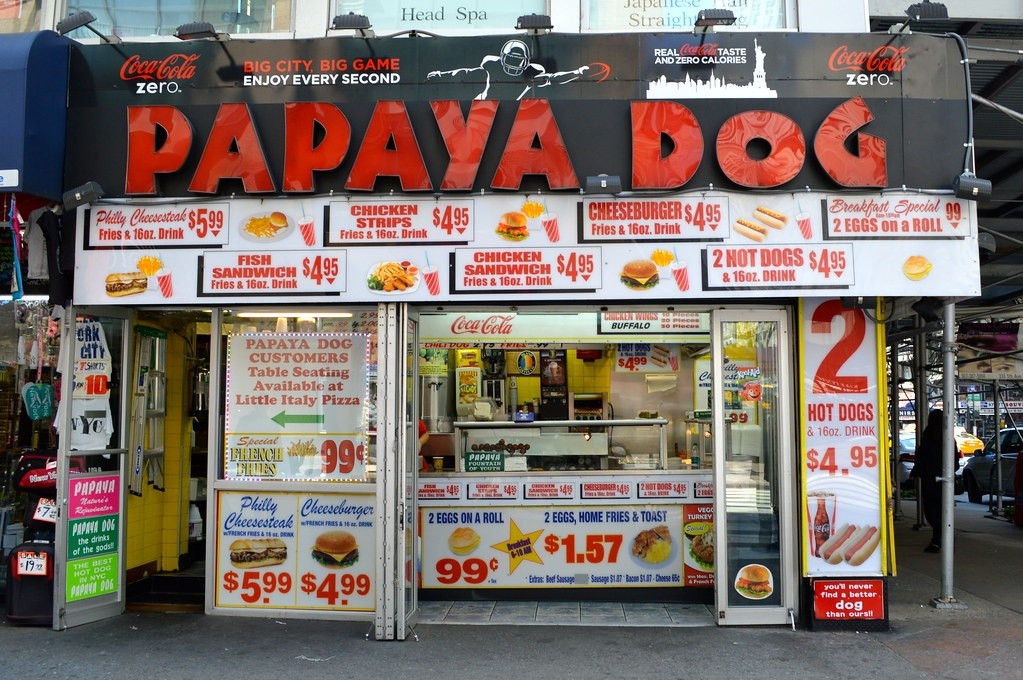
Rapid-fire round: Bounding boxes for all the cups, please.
[510,376,518,415]
[550,362,558,378]
[668,351,679,372]
[532,398,540,413]
[432,456,445,473]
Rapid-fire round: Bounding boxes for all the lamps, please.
[173,21,232,42]
[584,174,622,195]
[56,11,121,46]
[889,0,952,35]
[951,176,993,202]
[978,233,996,253]
[330,12,375,39]
[514,12,554,35]
[62,182,105,212]
[693,9,737,35]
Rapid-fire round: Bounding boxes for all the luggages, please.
[5,537,54,628]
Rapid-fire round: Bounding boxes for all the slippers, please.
[23,382,55,421]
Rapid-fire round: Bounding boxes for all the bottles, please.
[813,498,831,558]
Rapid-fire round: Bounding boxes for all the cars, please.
[953,426,985,455]
[898,432,970,490]
[963,427,1023,503]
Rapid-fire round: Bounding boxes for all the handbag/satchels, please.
[22,491,56,534]
[12,445,87,490]
[954,473,964,494]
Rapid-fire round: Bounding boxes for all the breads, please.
[270,212,289,227]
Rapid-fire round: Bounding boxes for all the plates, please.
[628,536,677,571]
[683,534,715,574]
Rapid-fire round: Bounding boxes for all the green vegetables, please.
[367,275,385,291]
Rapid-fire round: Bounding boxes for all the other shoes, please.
[924,542,940,553]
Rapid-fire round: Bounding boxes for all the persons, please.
[370,418,430,472]
[916,407,960,554]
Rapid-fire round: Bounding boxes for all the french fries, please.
[243,216,281,238]
[375,262,405,284]
[520,200,545,217]
[652,249,675,266]
[136,256,162,276]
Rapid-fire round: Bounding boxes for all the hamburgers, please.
[619,260,660,290]
[312,531,360,569]
[105,271,148,297]
[495,212,529,242]
[230,538,288,569]
[902,256,932,281]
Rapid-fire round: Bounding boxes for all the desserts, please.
[448,527,480,555]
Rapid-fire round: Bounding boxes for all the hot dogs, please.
[733,218,768,243]
[752,206,787,231]
[649,344,670,368]
[819,523,881,566]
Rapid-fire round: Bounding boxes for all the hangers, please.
[51,204,62,214]
